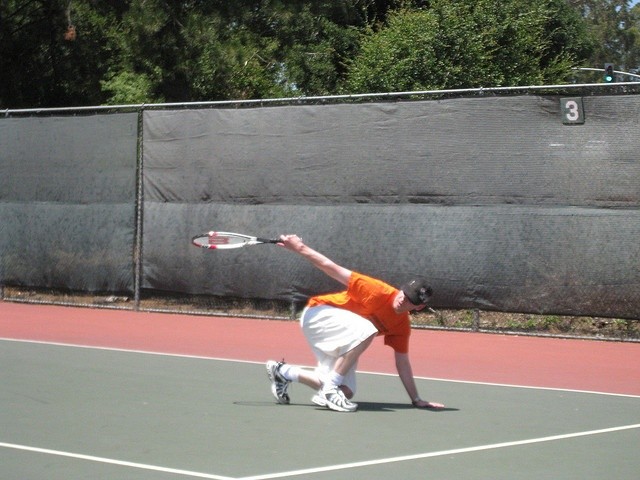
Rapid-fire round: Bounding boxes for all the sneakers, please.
[311,383,358,412]
[266,358,292,404]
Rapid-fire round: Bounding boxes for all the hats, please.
[401,275,434,305]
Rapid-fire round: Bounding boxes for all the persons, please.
[265,234,444,413]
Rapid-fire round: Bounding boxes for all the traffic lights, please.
[605,63,614,82]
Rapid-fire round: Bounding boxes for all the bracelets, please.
[412,396,422,403]
